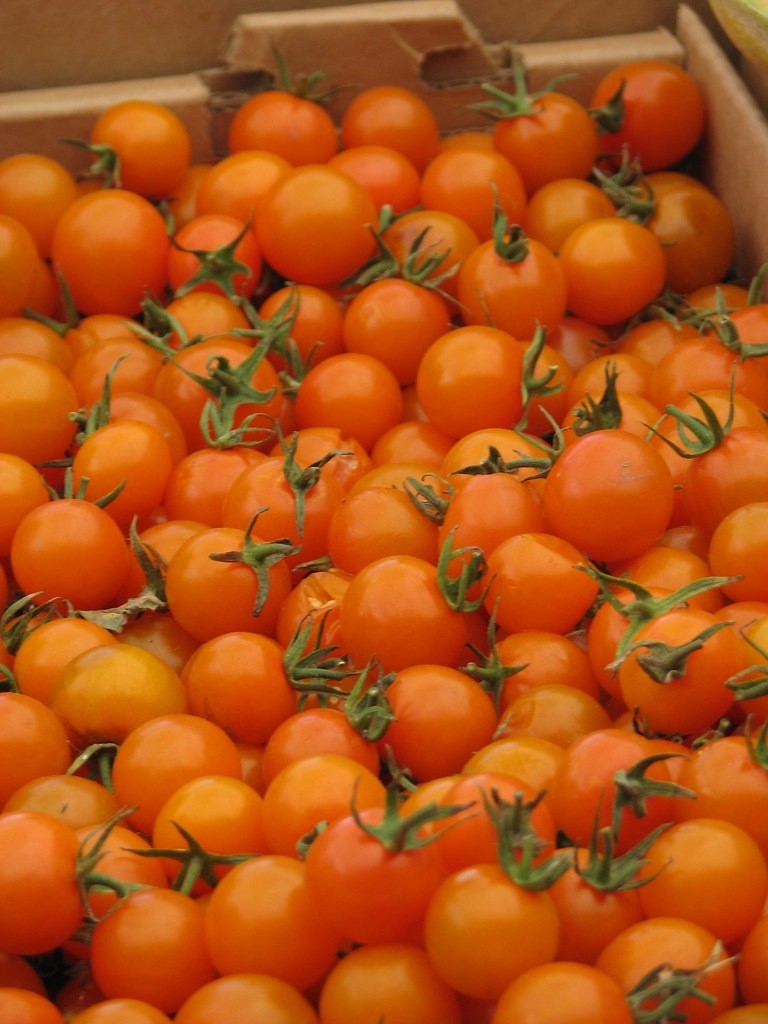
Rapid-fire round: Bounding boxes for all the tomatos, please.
[0,60,768,1024]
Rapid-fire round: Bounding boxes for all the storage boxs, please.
[0,0,767,1024]
